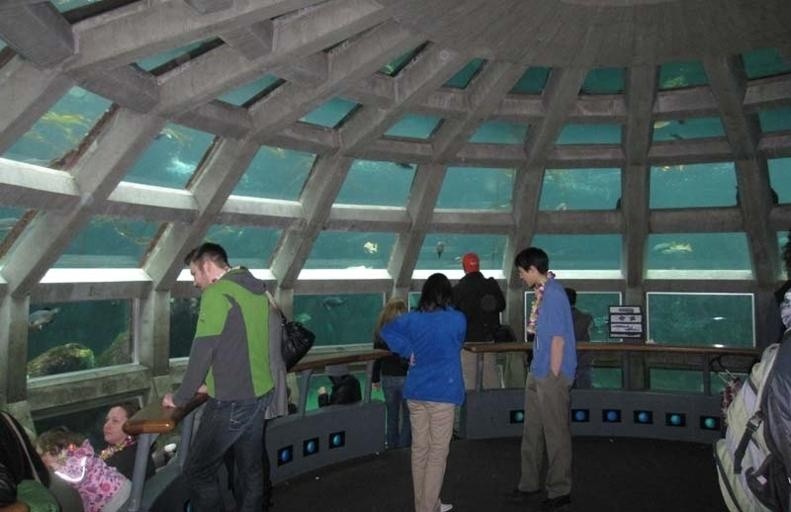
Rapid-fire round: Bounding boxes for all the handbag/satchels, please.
[281,318,315,371]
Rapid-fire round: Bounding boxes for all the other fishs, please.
[363,241,378,255]
[653,120,671,131]
[436,241,445,259]
[28,306,62,332]
[396,161,415,170]
[1,217,19,231]
[654,240,693,255]
[155,128,195,145]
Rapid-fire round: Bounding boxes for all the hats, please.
[463,253,479,272]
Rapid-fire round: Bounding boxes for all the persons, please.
[498,246,579,512]
[317,363,362,407]
[449,252,506,438]
[0,400,156,511]
[378,273,468,512]
[758,231,791,512]
[564,287,595,390]
[222,300,289,511]
[160,242,275,512]
[371,297,412,449]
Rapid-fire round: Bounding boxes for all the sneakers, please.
[498,489,545,505]
[437,499,454,512]
[537,493,575,510]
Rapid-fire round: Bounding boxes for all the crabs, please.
[39,109,95,132]
